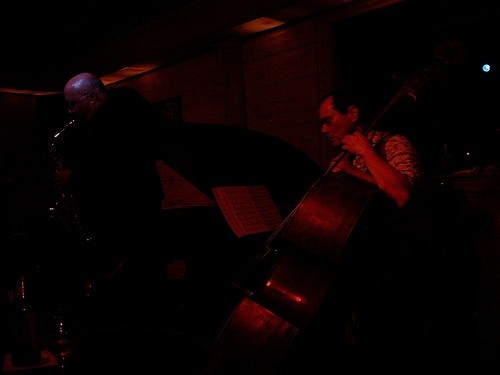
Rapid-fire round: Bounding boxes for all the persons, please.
[59,72,169,136]
[318,91,420,208]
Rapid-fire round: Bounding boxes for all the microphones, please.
[54,119,76,139]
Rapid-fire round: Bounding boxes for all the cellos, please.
[193,61,444,375]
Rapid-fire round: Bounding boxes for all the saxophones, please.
[43,107,97,255]
